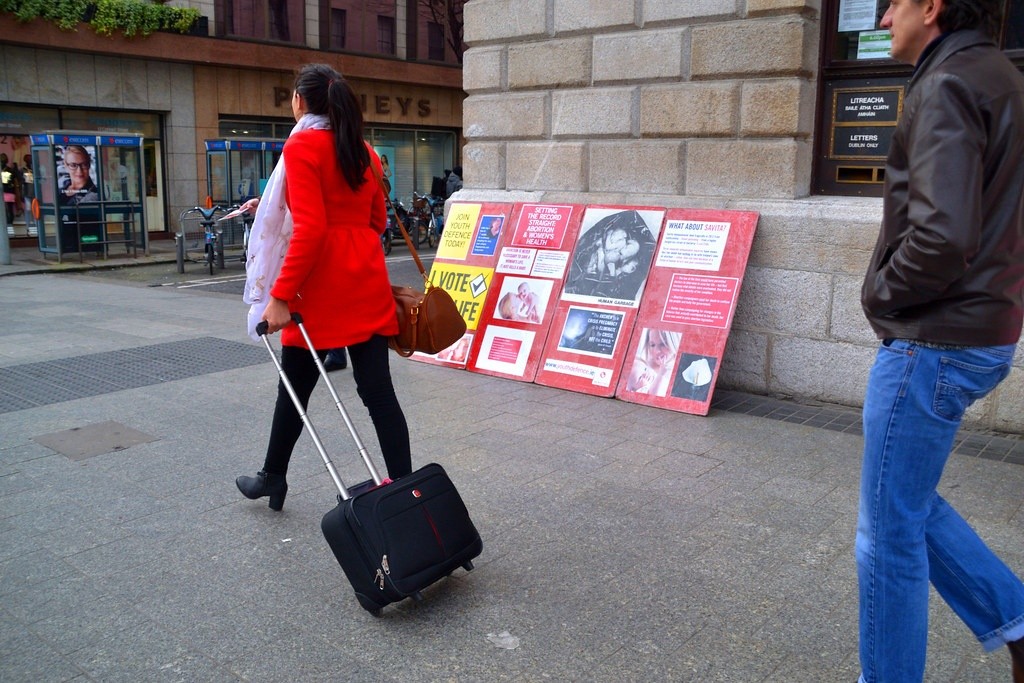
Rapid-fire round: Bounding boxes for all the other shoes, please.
[1006,635,1024,683]
[323,356,347,373]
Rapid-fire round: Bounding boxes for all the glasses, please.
[65,160,90,169]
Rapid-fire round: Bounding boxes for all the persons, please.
[488,219,501,237]
[498,292,536,323]
[19,155,31,196]
[439,338,470,362]
[628,328,680,395]
[58,145,97,204]
[381,155,391,194]
[11,162,24,217]
[518,283,540,319]
[446,166,462,196]
[855,1,1024,682]
[2,153,14,223]
[237,64,412,509]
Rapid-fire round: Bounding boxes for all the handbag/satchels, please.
[386,285,467,357]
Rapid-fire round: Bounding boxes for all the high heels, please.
[236,470,288,511]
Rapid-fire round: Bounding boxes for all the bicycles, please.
[185,204,225,276]
[381,191,444,256]
[227,203,255,270]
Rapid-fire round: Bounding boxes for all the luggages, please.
[256,311,483,618]
